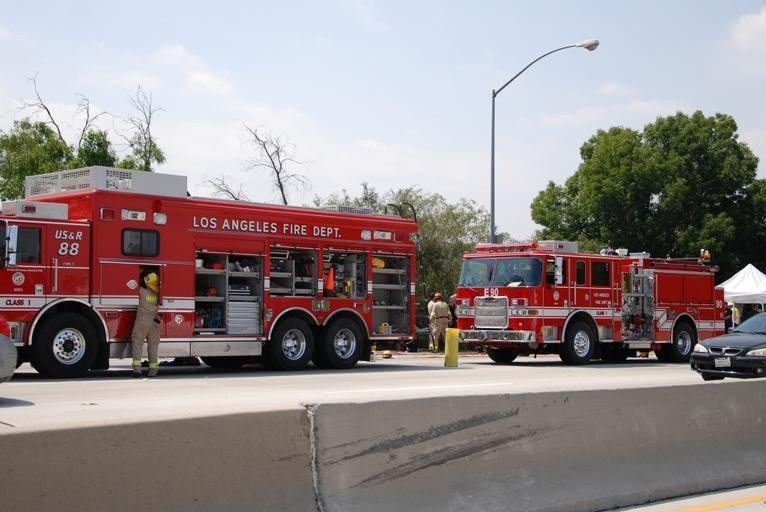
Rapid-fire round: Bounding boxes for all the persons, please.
[426,294,435,352]
[130,272,161,378]
[448,294,457,328]
[429,293,453,353]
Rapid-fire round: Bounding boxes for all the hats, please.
[143,272,159,292]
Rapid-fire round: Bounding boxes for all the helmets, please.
[434,292,441,299]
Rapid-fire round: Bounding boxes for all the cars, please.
[690,312,766,381]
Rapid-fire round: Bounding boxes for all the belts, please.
[435,315,449,319]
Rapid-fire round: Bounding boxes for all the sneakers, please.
[428,346,438,352]
[147,368,157,376]
[133,367,141,373]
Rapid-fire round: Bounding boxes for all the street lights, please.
[490,39,599,244]
